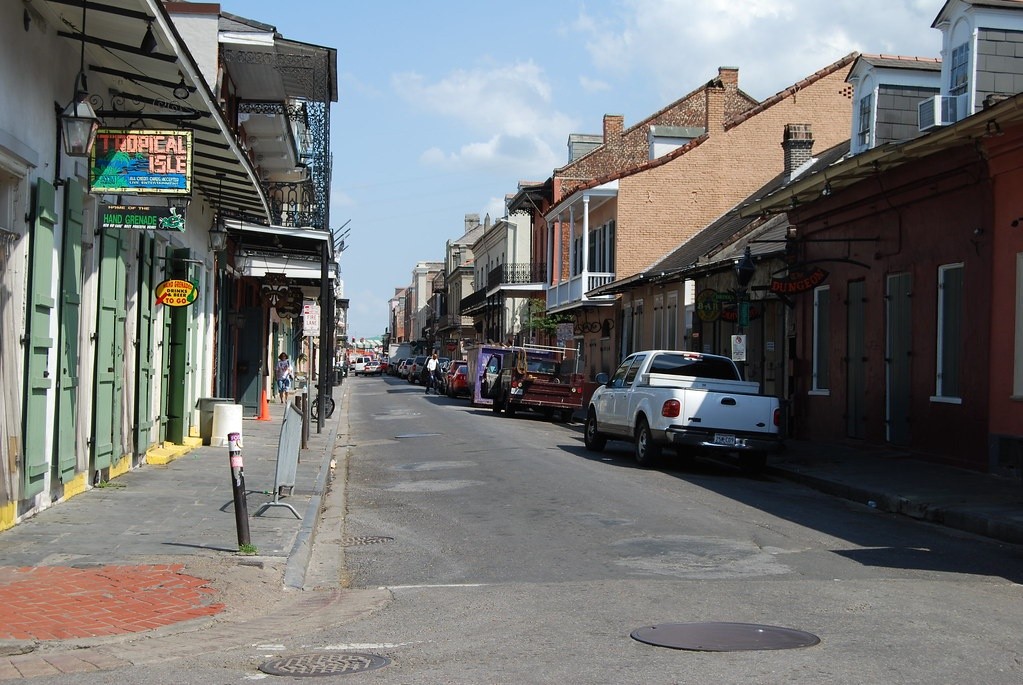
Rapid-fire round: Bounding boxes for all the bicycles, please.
[311,373,335,419]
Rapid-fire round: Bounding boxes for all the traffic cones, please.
[255,389,273,421]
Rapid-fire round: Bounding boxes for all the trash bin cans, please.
[195,397,243,448]
[342,364,348,377]
[333,366,342,385]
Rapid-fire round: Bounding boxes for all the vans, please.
[355,356,371,376]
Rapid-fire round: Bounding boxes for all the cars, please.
[388,356,471,399]
[350,359,355,371]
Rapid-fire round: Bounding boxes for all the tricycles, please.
[480,336,583,422]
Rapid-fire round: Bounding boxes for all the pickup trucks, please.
[585,349,781,467]
[364,361,382,376]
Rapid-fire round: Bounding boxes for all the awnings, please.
[649,250,787,286]
[585,266,683,298]
[740,90,1023,222]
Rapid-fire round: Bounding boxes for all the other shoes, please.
[285,400,288,403]
[434,391,440,394]
[281,400,283,403]
[425,391,430,394]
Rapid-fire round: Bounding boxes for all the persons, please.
[276,352,294,404]
[426,354,440,394]
